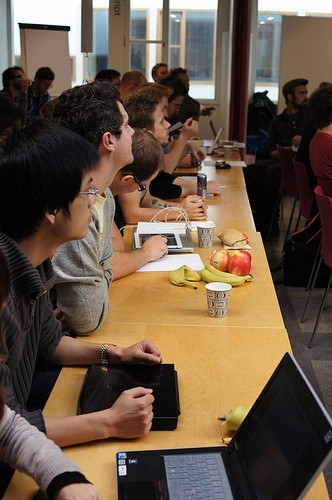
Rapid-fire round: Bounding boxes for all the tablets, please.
[133,231,183,249]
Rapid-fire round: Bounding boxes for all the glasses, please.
[120,173,146,192]
[76,185,99,200]
[42,80,53,89]
[171,101,182,108]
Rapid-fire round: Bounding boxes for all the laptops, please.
[115,349,332,500]
[209,119,239,147]
[188,126,224,154]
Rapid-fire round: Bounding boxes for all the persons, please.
[108,128,168,281]
[0,62,220,200]
[0,246,101,500]
[124,93,208,224]
[50,79,136,338]
[269,78,332,212]
[0,125,162,447]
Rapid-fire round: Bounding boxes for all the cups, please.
[205,282,232,318]
[197,221,216,248]
[224,146,233,159]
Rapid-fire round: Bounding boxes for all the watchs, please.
[100,343,117,373]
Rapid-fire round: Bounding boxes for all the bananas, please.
[200,264,253,286]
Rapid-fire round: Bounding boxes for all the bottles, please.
[191,152,201,168]
[197,173,206,200]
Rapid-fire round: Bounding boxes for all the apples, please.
[210,249,251,275]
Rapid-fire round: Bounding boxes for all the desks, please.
[0,140,332,500]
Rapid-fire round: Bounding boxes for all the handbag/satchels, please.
[75,362,181,431]
[283,241,332,289]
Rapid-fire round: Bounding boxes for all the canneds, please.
[197,174,207,198]
[191,153,198,167]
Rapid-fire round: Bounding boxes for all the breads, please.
[218,230,248,246]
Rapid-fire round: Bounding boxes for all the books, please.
[174,161,201,173]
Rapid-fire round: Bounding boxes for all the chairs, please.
[300,185,332,349]
[293,158,315,232]
[274,144,299,253]
